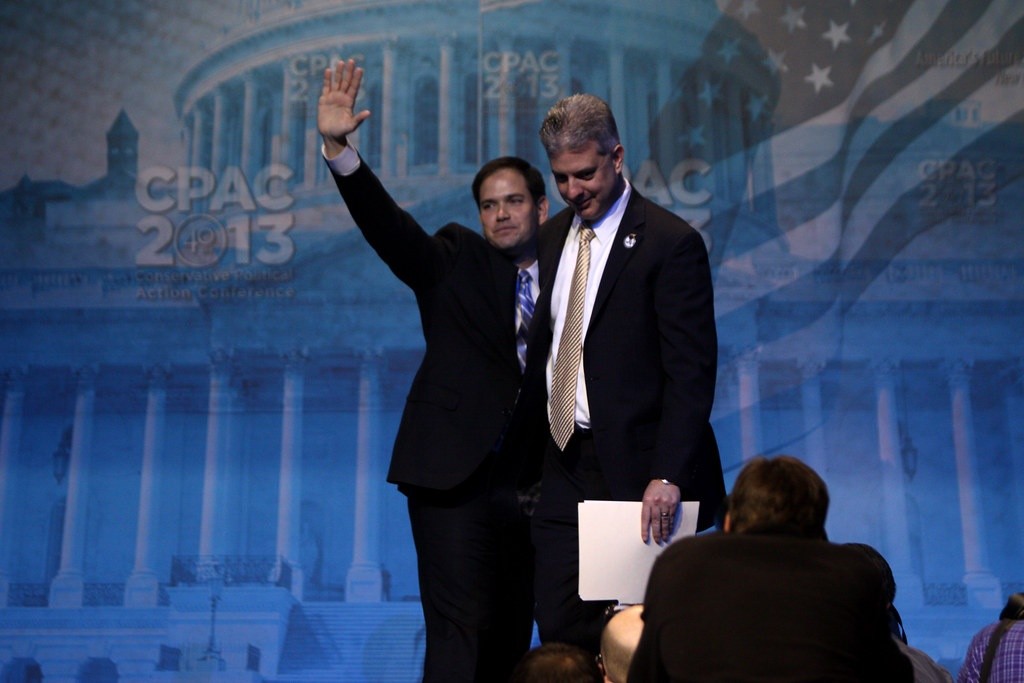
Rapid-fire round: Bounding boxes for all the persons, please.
[626,453,917,683]
[511,645,605,683]
[598,605,646,683]
[538,94,725,644]
[955,619,1024,682]
[316,59,547,683]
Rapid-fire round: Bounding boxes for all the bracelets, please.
[661,479,671,485]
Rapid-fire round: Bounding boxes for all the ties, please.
[548,220,595,456]
[514,269,535,372]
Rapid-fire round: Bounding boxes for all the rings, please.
[662,512,669,516]
